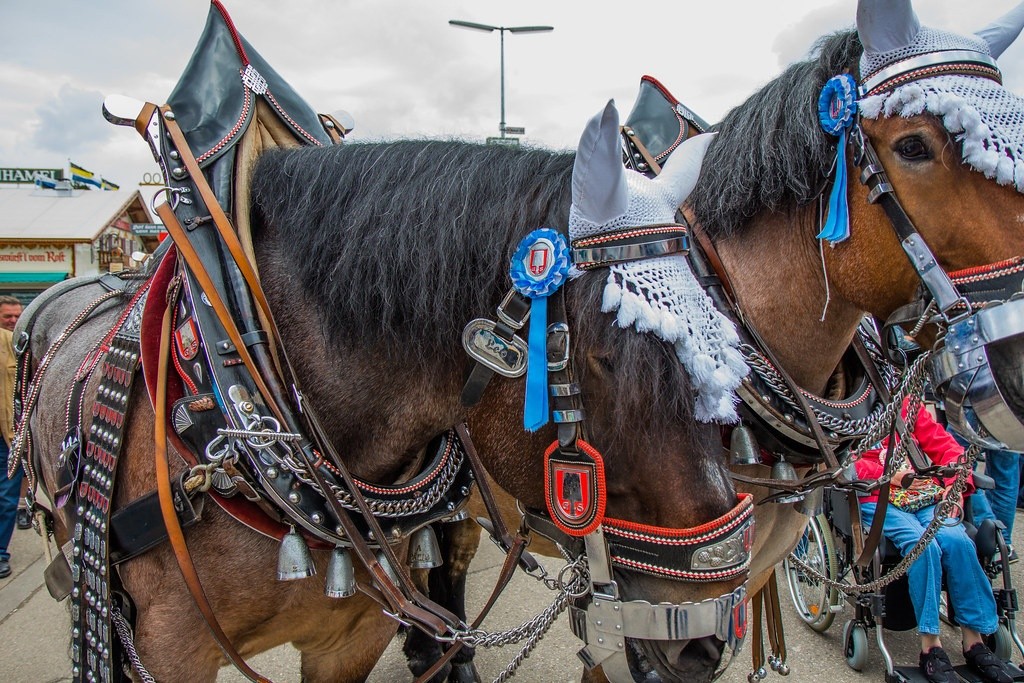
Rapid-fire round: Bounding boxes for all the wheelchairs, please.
[783,470,1024,683]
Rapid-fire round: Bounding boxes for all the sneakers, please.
[919,647,961,683]
[962,640,1014,683]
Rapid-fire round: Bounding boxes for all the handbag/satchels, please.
[887,473,946,513]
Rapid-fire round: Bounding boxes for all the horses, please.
[11,0,1024,682]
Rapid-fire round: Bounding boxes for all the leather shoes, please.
[17,508,32,529]
[0,561,11,578]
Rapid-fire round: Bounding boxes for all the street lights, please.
[449,20,554,147]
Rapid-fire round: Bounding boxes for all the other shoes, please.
[1006,549,1019,564]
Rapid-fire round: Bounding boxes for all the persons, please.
[0,294,31,579]
[844,380,1024,682]
